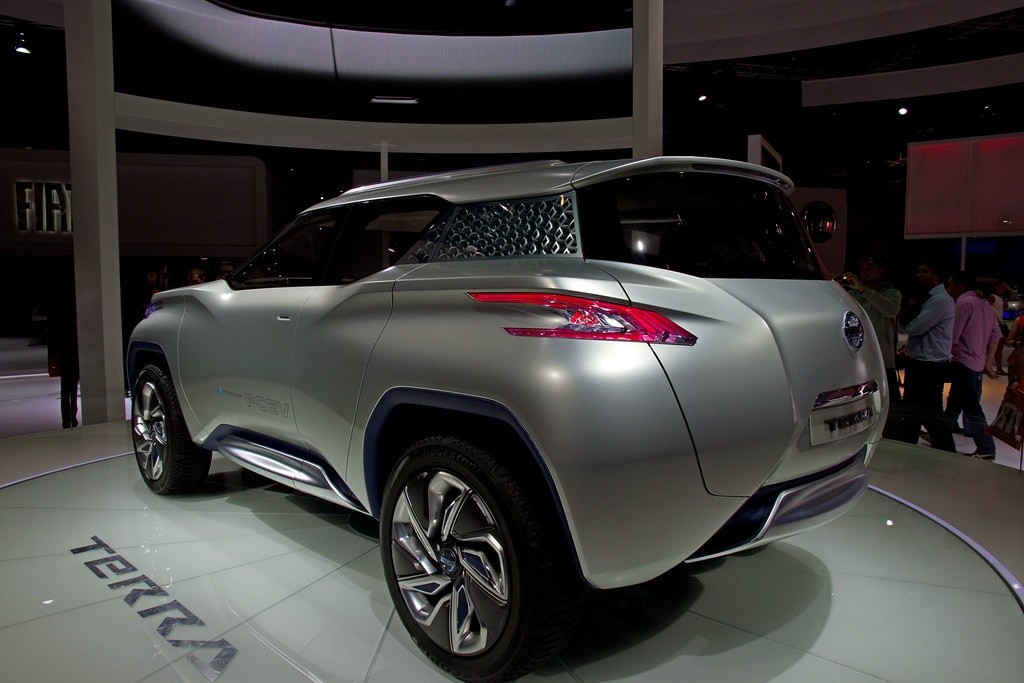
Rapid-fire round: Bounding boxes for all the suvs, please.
[127,153,892,683]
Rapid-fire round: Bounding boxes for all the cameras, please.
[220,270,234,279]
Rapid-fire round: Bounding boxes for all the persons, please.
[49,261,232,430]
[835,252,1024,464]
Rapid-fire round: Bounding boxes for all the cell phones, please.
[834,274,852,285]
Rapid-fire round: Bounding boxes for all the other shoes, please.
[952,425,964,434]
[964,449,995,461]
[920,430,931,442]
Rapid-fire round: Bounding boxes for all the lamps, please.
[14,29,33,55]
